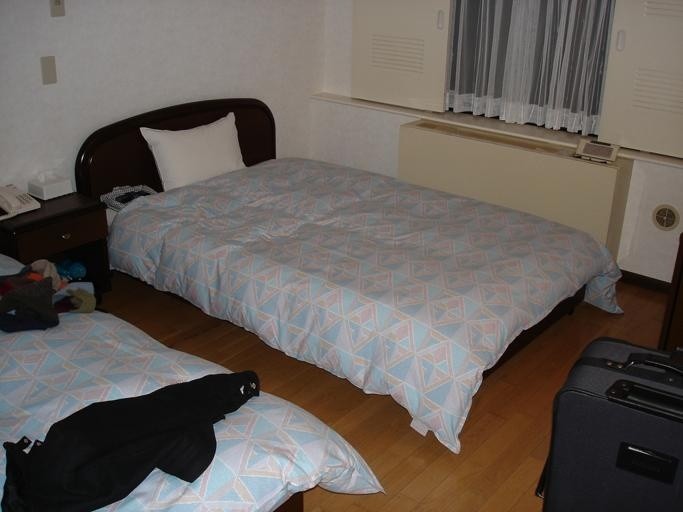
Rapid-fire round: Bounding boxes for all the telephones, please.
[0,184,41,221]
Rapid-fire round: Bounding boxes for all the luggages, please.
[535,338,683,512]
